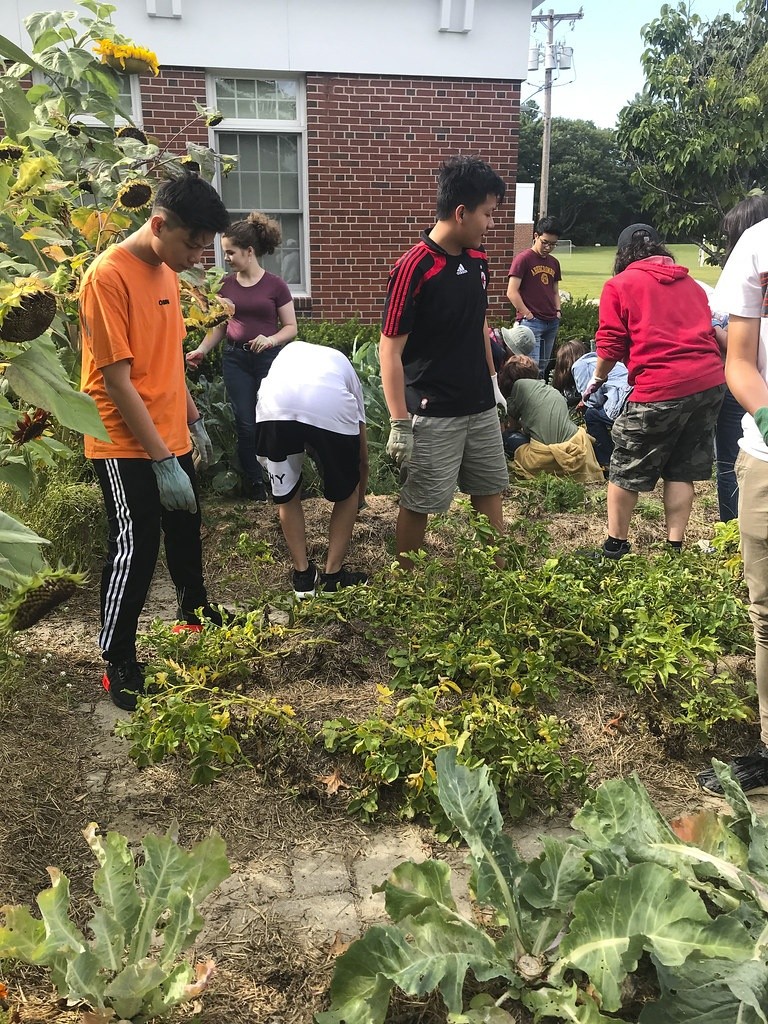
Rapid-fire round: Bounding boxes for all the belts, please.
[228,338,251,351]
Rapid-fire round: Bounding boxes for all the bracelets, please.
[523,311,531,318]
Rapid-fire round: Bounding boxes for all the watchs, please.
[557,310,562,313]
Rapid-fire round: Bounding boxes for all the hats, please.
[618,223,660,251]
[501,325,536,355]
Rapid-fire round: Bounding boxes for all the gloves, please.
[151,453,197,514]
[386,418,414,485]
[491,372,507,418]
[187,413,213,472]
[583,369,608,402]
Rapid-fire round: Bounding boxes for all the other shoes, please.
[698,539,715,553]
[250,489,268,504]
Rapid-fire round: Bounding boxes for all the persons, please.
[254,340,371,599]
[694,217,768,799]
[489,194,768,523]
[185,212,299,505]
[77,175,245,711]
[379,158,511,598]
[574,223,728,567]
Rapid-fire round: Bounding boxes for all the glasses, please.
[539,237,558,248]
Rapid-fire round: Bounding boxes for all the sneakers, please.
[574,542,631,562]
[176,603,247,630]
[662,547,681,558]
[321,566,368,598]
[293,561,318,599]
[102,661,145,711]
[695,747,768,796]
